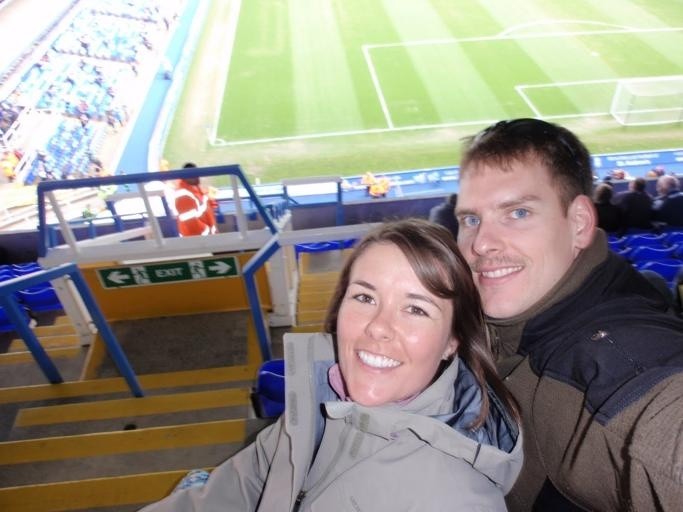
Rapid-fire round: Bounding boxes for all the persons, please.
[173,163,219,237]
[131,216,527,512]
[455,117,682,511]
[426,193,459,240]
[588,166,683,233]
[340,171,455,199]
[0,1,178,219]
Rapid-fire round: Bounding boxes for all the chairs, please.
[0,261,60,334]
[605,228,683,295]
